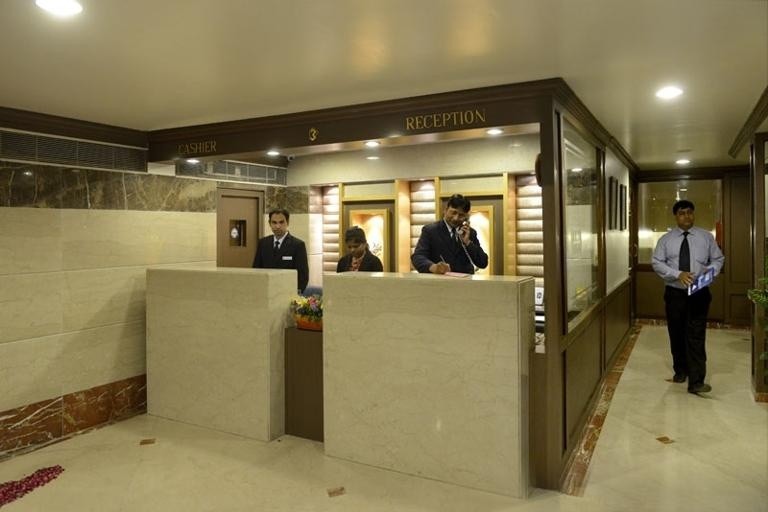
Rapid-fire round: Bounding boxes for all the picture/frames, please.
[535,287,544,306]
[609,175,618,230]
[567,167,597,206]
[348,208,389,271]
[619,183,627,231]
[468,206,494,276]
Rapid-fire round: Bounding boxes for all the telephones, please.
[456,220,470,235]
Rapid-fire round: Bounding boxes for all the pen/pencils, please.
[439,254,445,264]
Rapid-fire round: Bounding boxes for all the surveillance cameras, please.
[287,153,296,163]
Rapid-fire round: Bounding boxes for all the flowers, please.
[289,294,323,322]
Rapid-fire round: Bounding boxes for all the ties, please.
[451,227,458,253]
[273,240,280,257]
[679,231,691,274]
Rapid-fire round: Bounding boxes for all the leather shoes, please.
[687,382,711,392]
[673,372,688,383]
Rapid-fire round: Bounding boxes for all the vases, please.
[294,316,322,331]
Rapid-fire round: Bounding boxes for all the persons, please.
[336,226,383,273]
[250,208,308,294]
[410,194,488,275]
[652,199,725,395]
